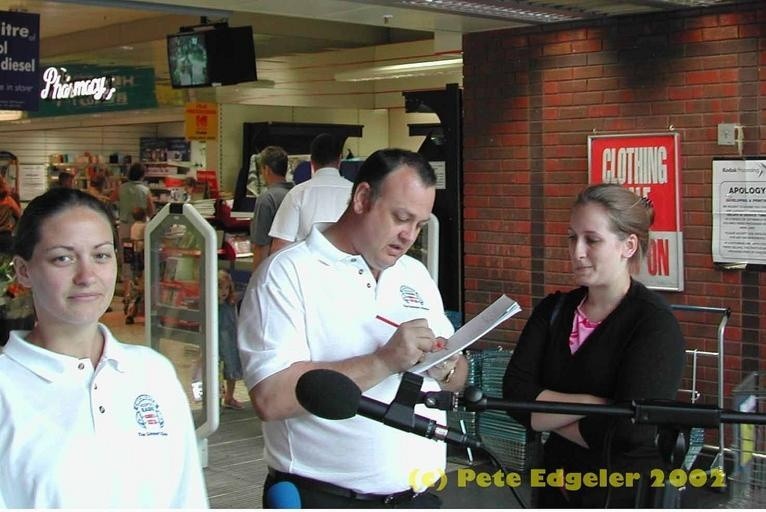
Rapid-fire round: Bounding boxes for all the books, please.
[407,293,523,376]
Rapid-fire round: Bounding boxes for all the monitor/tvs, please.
[166,14,259,88]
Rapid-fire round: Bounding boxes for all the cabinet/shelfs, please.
[44,159,193,298]
[139,200,253,440]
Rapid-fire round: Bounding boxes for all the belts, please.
[266,465,430,503]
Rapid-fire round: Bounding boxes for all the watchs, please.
[443,368,456,385]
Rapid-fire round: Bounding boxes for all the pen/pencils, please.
[376,314,450,352]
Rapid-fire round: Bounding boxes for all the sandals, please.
[223,396,245,410]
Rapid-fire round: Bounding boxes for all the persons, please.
[130,206,146,251]
[201,270,246,411]
[59,171,75,191]
[250,146,295,273]
[0,185,210,508]
[236,147,469,508]
[266,133,355,258]
[116,162,155,325]
[85,173,111,204]
[502,184,687,508]
[181,177,196,202]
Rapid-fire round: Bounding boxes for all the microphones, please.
[295,368,480,448]
[267,481,300,508]
